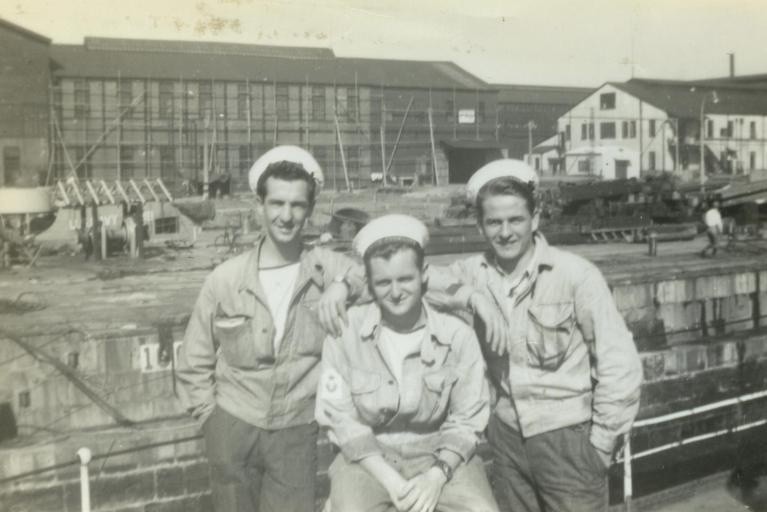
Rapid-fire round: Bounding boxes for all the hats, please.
[247,143,326,203]
[351,212,432,257]
[462,158,540,210]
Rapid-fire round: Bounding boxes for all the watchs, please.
[330,270,350,293]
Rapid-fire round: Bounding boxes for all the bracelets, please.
[430,458,452,482]
[470,286,485,316]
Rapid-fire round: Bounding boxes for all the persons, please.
[418,156,646,511]
[308,211,500,510]
[171,144,369,512]
[700,199,723,259]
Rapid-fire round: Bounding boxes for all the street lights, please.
[175,89,196,169]
[262,69,279,148]
[698,88,722,193]
[214,112,226,172]
